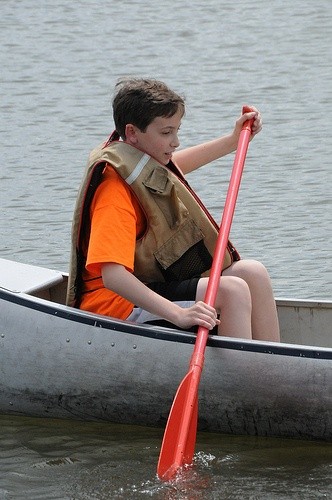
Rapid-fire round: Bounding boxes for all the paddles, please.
[156,104,257,481]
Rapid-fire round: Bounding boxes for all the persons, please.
[64,79,281,342]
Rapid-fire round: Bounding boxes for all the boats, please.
[0,256,332,442]
